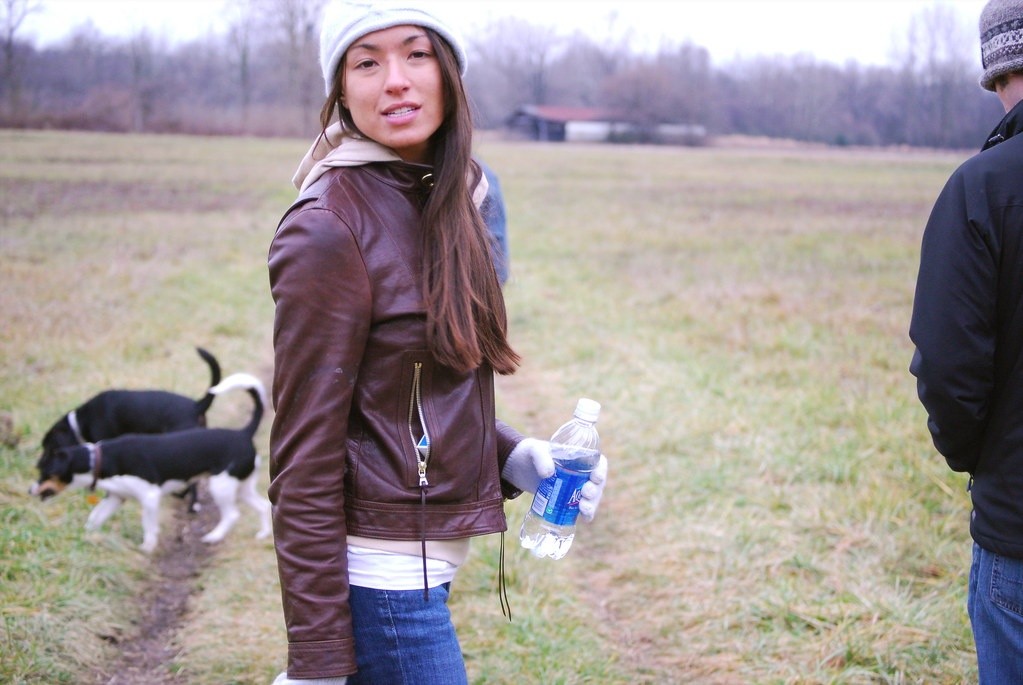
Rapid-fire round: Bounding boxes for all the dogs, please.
[26,371,272,554]
[35,344,222,516]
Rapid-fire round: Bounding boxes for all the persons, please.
[267,0,608,685]
[909,0,1023,685]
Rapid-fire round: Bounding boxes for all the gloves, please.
[502,438,607,522]
[273,673,347,685]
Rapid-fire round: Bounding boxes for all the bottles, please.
[520,398,601,560]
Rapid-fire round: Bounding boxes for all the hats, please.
[979,0,1023,92]
[318,0,468,106]
